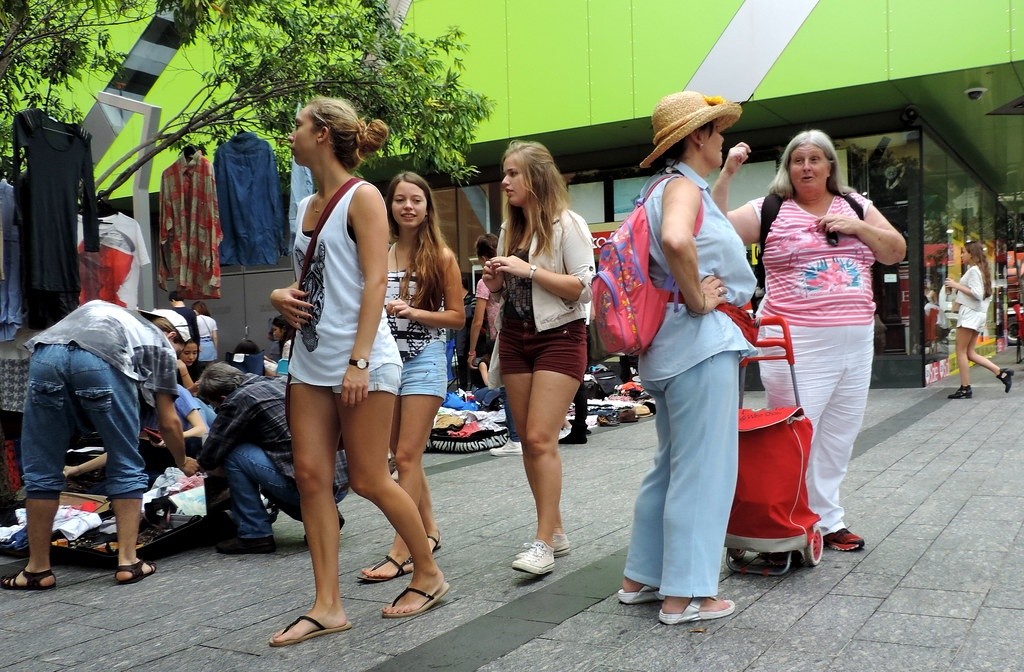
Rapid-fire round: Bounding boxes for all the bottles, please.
[945,279,952,295]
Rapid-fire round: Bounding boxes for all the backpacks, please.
[591,174,703,356]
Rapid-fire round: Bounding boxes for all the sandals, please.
[0,562,57,591]
[115,559,157,585]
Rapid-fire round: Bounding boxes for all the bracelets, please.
[175,458,187,467]
[469,350,475,355]
[688,290,706,312]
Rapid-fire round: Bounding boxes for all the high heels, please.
[948,385,972,399]
[996,367,1014,393]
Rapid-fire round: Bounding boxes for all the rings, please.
[716,287,724,294]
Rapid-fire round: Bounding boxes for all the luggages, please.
[724,316,823,577]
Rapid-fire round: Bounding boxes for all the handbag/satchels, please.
[285,373,345,451]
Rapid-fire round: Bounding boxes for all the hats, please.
[619,401,656,423]
[639,91,743,169]
[136,308,191,342]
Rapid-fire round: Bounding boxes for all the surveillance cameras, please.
[964,88,988,102]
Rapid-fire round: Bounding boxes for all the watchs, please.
[529,264,537,278]
[348,359,369,370]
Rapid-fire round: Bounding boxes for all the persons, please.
[712,128,908,553]
[268,93,449,647]
[193,360,350,554]
[355,170,465,582]
[619,91,759,625]
[943,240,1014,399]
[62,291,296,496]
[0,300,199,591]
[456,139,597,574]
[924,275,939,353]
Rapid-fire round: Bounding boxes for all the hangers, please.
[78,189,118,225]
[180,128,197,162]
[235,116,249,136]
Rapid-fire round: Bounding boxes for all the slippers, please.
[617,585,665,604]
[382,582,450,618]
[269,615,352,647]
[658,596,735,625]
[428,529,442,554]
[356,554,414,581]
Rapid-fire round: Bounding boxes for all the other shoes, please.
[558,425,589,444]
[214,535,276,554]
[304,510,345,543]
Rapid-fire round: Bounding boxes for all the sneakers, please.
[551,533,570,558]
[824,528,865,551]
[490,438,524,457]
[511,539,555,575]
